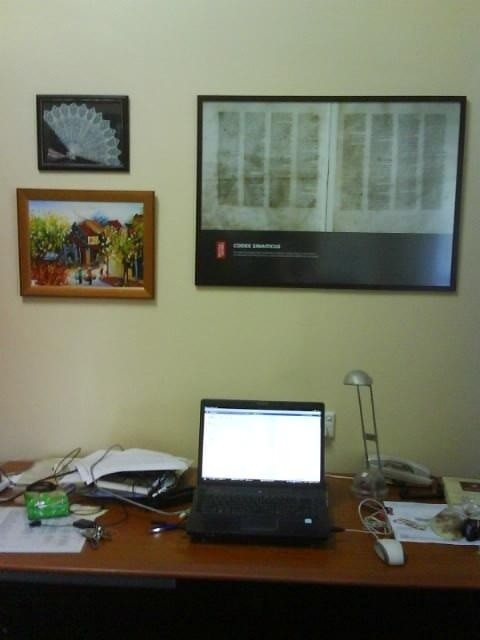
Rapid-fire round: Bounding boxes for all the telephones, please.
[368,455,432,485]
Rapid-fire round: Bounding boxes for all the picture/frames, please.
[17,188,155,299]
[194,95,466,292]
[35,94,129,171]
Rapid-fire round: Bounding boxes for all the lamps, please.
[343,370,389,502]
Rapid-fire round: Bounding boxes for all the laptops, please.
[188,399,331,541]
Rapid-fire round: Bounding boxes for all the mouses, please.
[374,537,408,566]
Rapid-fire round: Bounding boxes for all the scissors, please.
[148,520,184,533]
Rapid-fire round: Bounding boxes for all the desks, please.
[1,461,480,640]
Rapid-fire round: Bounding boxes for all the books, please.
[14,455,89,489]
[94,478,194,498]
[382,499,480,547]
[439,475,480,509]
